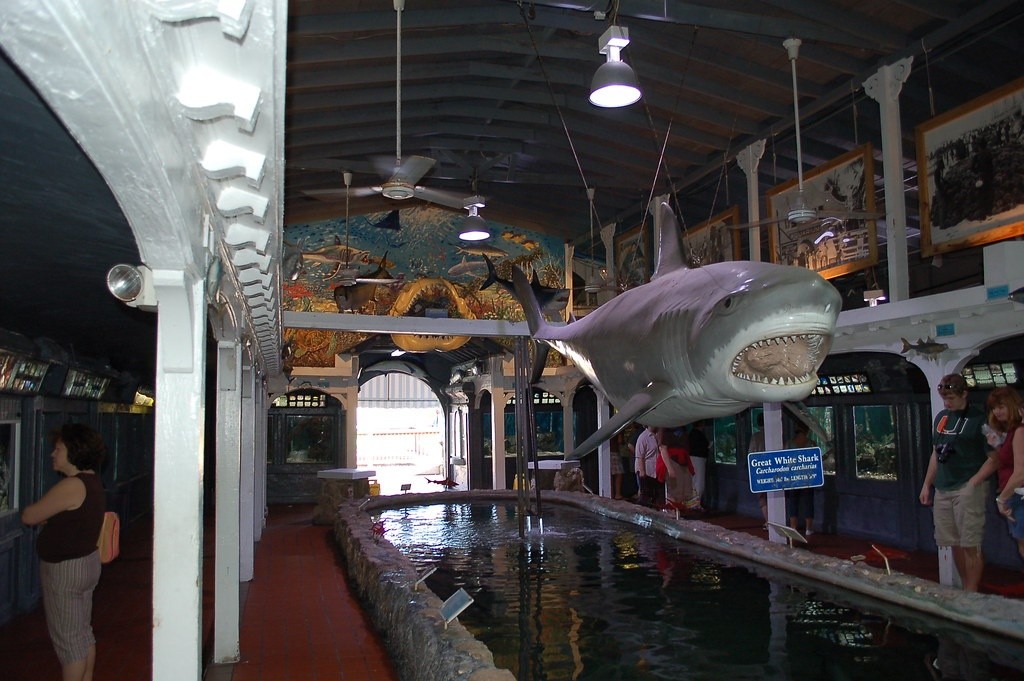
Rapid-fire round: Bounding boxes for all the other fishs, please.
[900,334,951,362]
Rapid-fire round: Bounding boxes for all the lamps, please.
[458,193,491,243]
[861,286,887,308]
[587,23,643,108]
[106,259,156,309]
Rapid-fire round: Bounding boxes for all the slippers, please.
[617,496,628,501]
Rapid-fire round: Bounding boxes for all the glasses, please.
[938,384,960,390]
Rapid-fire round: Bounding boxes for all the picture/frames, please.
[683,201,743,268]
[913,73,1024,260]
[765,139,879,281]
[615,220,652,291]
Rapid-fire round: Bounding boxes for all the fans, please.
[302,165,403,288]
[302,0,466,217]
[543,183,640,297]
[721,37,888,234]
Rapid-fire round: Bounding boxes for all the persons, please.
[749,414,769,530]
[656,420,710,511]
[635,426,663,506]
[986,387,1024,557]
[610,429,638,500]
[785,421,817,535]
[23,425,105,681]
[920,374,999,593]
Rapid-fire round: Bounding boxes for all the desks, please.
[316,466,378,501]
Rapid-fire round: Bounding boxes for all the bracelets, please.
[995,497,1007,505]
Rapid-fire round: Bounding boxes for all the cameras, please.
[935,443,956,465]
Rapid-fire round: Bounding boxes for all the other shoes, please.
[762,523,768,531]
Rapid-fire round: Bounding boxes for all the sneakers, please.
[806,530,813,535]
